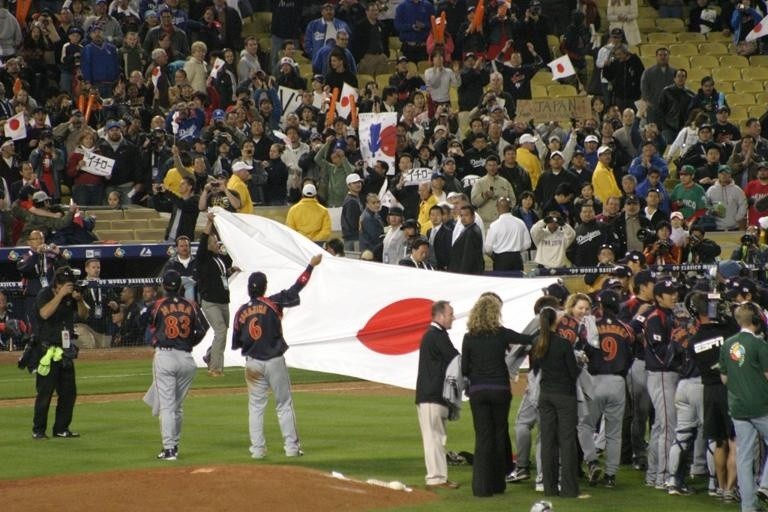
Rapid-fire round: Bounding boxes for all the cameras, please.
[358,160,364,166]
[156,187,162,191]
[742,232,759,246]
[211,183,219,187]
[691,235,700,243]
[71,280,89,295]
[636,227,657,245]
[43,245,52,252]
[335,137,346,149]
[18,333,37,369]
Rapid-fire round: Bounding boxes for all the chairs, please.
[0,0,768,260]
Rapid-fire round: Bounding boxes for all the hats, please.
[541,251,756,307]
[18,184,53,204]
[396,55,409,63]
[346,173,364,185]
[310,132,323,142]
[213,109,225,121]
[107,119,121,130]
[164,270,181,286]
[431,139,465,182]
[302,183,317,197]
[386,205,418,230]
[464,51,477,60]
[249,272,267,289]
[232,161,254,172]
[625,106,767,221]
[518,134,614,160]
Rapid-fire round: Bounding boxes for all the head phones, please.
[690,294,698,320]
[743,301,761,325]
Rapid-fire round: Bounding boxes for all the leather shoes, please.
[430,479,461,489]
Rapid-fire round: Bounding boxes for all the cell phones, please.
[490,186,493,191]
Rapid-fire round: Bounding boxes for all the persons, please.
[144,270,207,460]
[415,300,466,489]
[504,250,768,511]
[280,0,694,277]
[1,0,290,247]
[461,292,540,498]
[31,267,92,436]
[1,229,198,351]
[195,212,241,377]
[649,1,768,263]
[231,253,323,460]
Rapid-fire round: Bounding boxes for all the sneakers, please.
[505,459,616,499]
[633,456,767,505]
[155,445,177,461]
[32,430,49,440]
[53,431,80,438]
